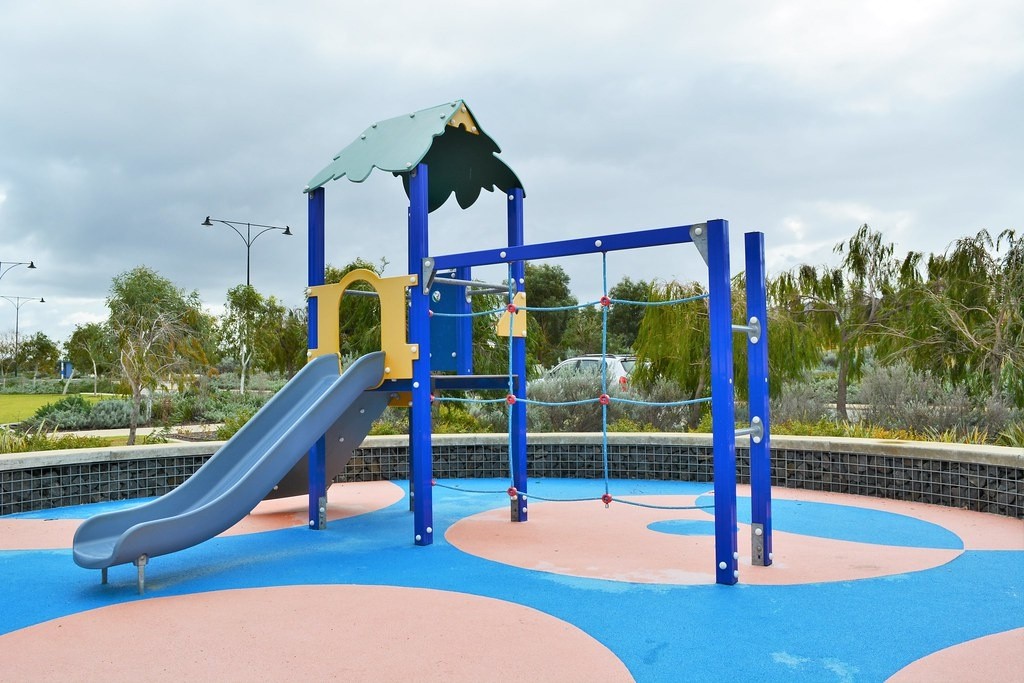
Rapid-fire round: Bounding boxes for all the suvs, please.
[525,353,663,401]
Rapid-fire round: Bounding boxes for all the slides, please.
[72,351,385,570]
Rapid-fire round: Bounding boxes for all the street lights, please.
[200,215,293,358]
[1,294,47,377]
